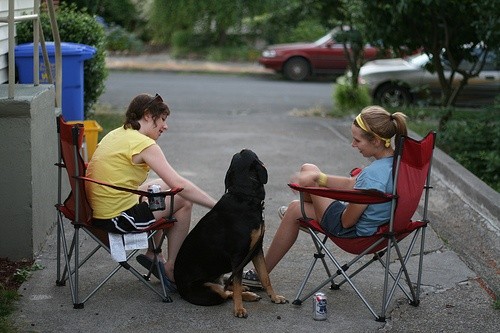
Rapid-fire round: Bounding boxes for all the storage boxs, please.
[65,119,102,161]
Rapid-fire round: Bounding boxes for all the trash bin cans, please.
[14,41,99,120]
[66,121,103,162]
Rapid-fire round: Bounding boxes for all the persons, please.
[243,105,408,287]
[86,95,217,290]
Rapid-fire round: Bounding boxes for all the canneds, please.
[313,292,328,321]
[147,184,166,210]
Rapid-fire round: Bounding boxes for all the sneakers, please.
[241,269,263,287]
[278,206,318,234]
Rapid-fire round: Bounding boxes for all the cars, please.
[256,25,425,82]
[357,41,500,113]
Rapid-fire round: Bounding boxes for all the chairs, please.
[287,130,438,322]
[54,115,184,310]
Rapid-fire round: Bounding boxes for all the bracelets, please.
[319,172,327,185]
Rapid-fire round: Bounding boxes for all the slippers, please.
[159,260,179,292]
[136,253,165,280]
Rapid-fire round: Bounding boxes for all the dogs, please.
[172,147,290,319]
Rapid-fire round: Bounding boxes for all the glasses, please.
[142,93,163,113]
